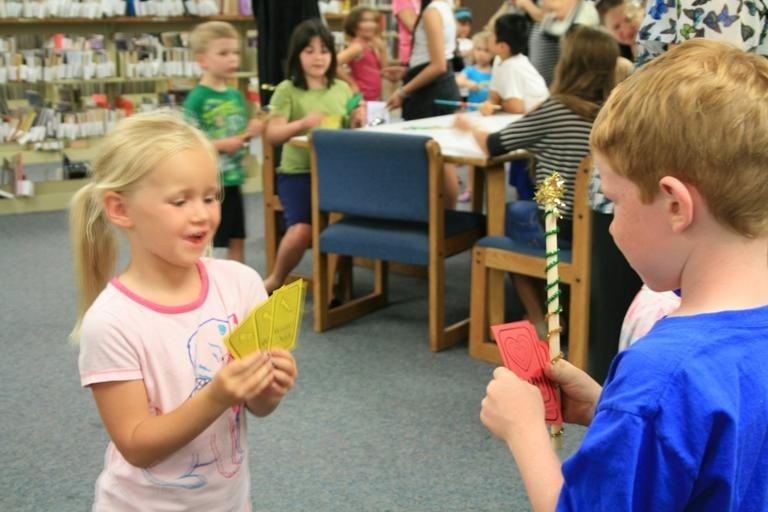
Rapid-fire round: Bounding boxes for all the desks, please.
[289,111,527,342]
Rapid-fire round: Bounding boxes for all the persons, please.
[509,1,599,90]
[455,8,474,103]
[456,33,494,202]
[479,38,768,512]
[588,1,767,354]
[596,1,645,81]
[180,21,265,263]
[261,20,368,295]
[379,1,465,121]
[454,26,617,341]
[481,14,551,200]
[636,0,766,71]
[68,111,299,512]
[251,1,326,114]
[391,1,422,66]
[336,7,389,101]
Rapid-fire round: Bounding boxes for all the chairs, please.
[255,103,352,302]
[308,127,486,351]
[469,156,593,372]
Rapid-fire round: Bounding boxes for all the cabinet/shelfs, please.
[0,0,399,216]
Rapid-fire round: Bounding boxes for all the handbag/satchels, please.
[402,11,457,121]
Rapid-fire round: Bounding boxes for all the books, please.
[0,0,253,18]
[319,1,378,16]
[0,94,180,151]
[0,33,206,76]
[0,153,35,199]
